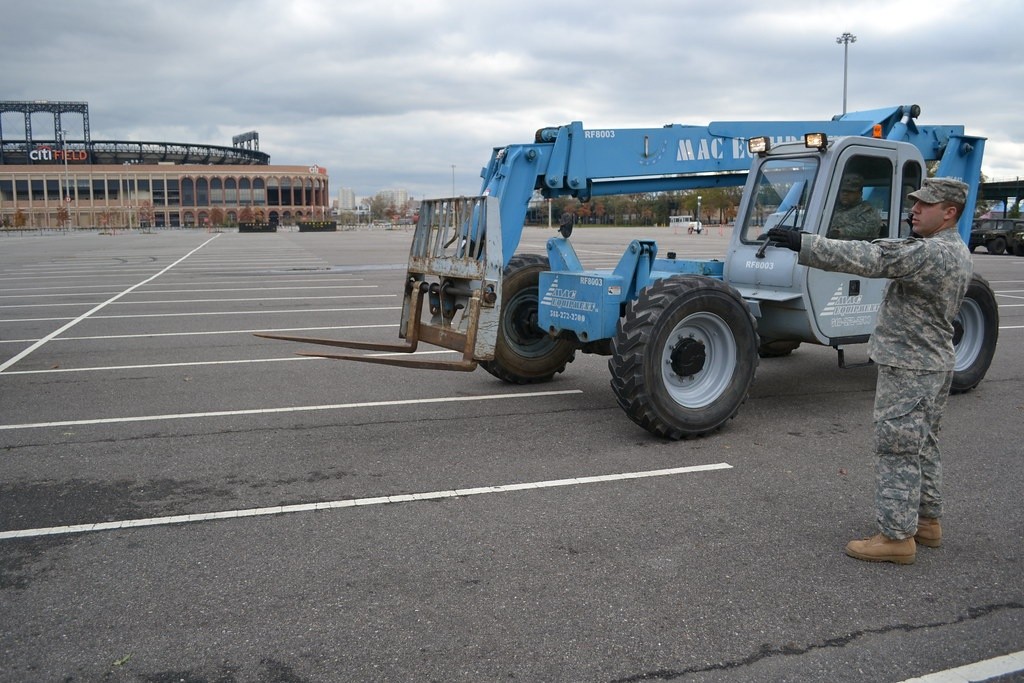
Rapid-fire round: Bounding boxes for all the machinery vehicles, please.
[252,105,999,441]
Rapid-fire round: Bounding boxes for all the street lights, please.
[451,164,456,197]
[57,130,71,232]
[836,32,857,114]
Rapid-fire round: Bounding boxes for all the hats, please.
[840,174,864,191]
[907,178,969,204]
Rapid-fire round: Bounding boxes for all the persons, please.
[830,172,881,240]
[768,176,974,565]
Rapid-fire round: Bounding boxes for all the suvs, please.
[969,218,1024,255]
[1011,230,1024,257]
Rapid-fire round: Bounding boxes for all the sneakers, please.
[845,532,916,564]
[914,517,942,547]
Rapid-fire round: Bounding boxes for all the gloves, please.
[827,230,840,239]
[767,228,802,251]
